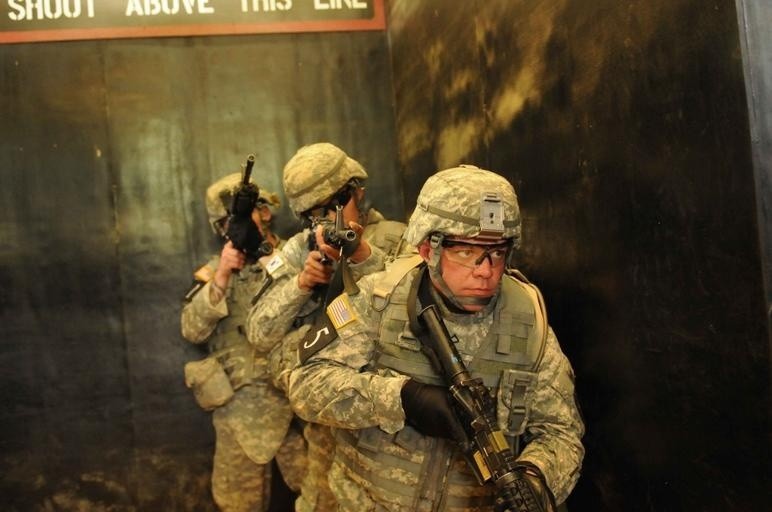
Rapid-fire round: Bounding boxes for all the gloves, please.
[401,380,476,440]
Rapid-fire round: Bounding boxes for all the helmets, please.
[281,142,368,221]
[206,172,280,235]
[401,164,522,251]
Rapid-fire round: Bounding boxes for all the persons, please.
[178,173,308,512]
[289,163,586,512]
[245,141,408,512]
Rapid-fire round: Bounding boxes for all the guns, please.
[476,393,487,397]
[408,305,549,511]
[222,155,273,275]
[305,204,356,287]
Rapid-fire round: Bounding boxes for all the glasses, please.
[427,237,513,268]
[301,185,353,217]
